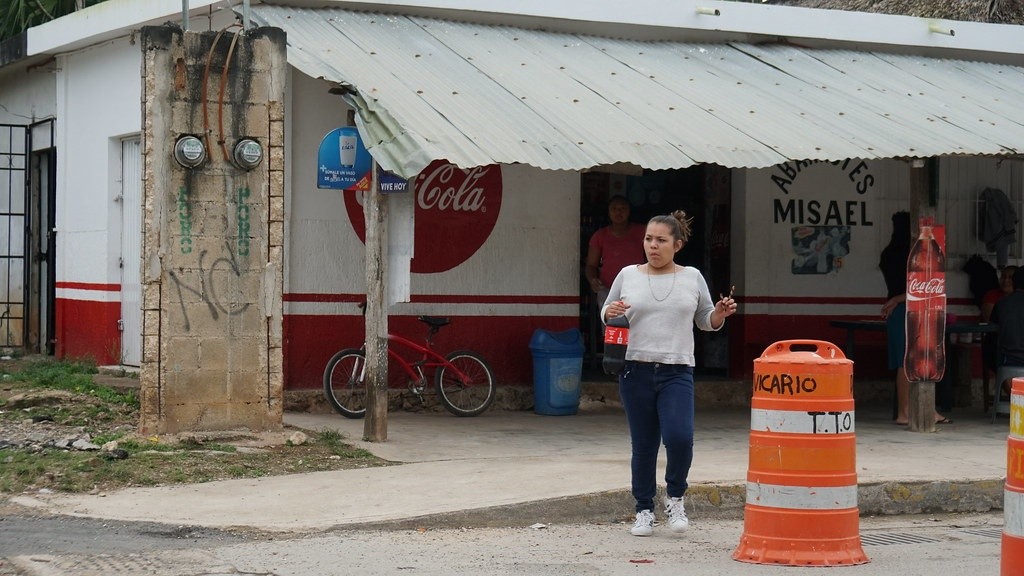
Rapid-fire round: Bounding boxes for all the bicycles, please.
[323,301,497,419]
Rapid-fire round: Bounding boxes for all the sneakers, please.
[631,509,655,536]
[663,490,689,533]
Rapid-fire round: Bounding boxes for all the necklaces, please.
[645,260,676,302]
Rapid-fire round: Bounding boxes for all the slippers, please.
[897,422,909,425]
[935,417,954,424]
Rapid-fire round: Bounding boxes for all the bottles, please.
[903,217,946,383]
[603,301,629,376]
[596,285,610,311]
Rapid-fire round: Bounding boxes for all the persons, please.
[586,195,647,382]
[982,263,1024,421]
[880,210,954,427]
[597,208,738,537]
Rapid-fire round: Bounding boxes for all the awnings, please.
[234,5,1024,178]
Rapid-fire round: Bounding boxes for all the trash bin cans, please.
[528,327,588,416]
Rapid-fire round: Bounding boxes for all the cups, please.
[339,130,357,168]
[946,313,956,324]
